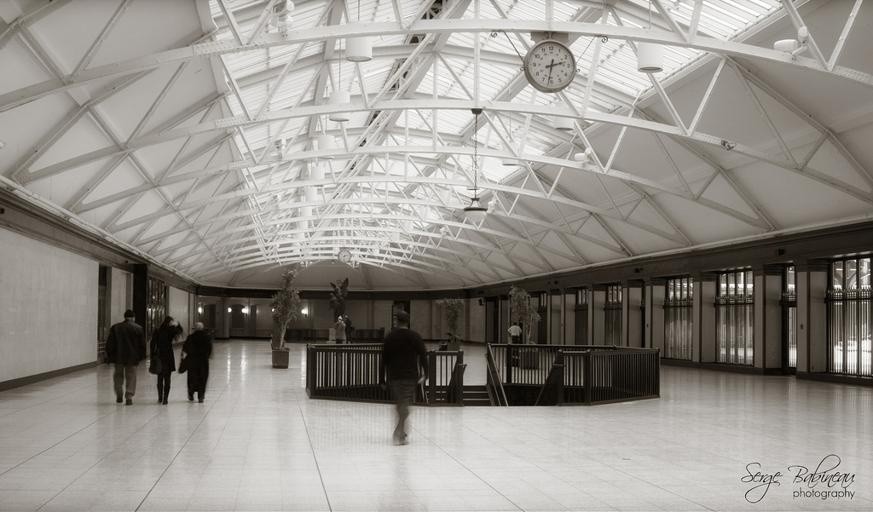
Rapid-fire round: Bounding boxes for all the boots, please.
[162,386,171,405]
[157,383,162,404]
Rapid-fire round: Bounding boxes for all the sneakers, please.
[115,395,133,405]
[393,432,409,445]
[187,394,205,403]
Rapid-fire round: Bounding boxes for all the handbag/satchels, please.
[148,351,162,374]
[178,357,186,373]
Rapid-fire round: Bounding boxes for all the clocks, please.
[337,249,352,264]
[524,38,577,95]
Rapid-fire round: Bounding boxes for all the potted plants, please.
[508,284,543,370]
[442,296,461,351]
[326,274,349,342]
[269,260,302,370]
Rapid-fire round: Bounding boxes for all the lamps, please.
[500,113,520,166]
[553,92,576,133]
[462,106,488,225]
[632,1,667,75]
[297,0,374,239]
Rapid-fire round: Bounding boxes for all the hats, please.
[123,309,134,317]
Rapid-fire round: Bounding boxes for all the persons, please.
[343,315,353,343]
[106,309,148,405]
[508,322,522,343]
[334,315,346,344]
[379,310,428,445]
[183,322,212,402]
[149,316,183,405]
